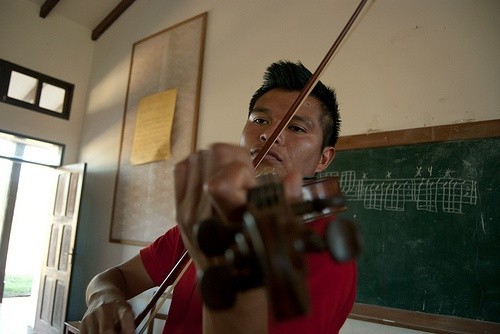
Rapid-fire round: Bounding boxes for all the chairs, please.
[148,292,171,334]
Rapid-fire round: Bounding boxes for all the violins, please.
[194,177,357,319]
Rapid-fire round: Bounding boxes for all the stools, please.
[63,319,83,334]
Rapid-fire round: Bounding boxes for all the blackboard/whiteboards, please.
[314,118,500,334]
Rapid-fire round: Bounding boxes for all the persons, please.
[78,59,359,334]
